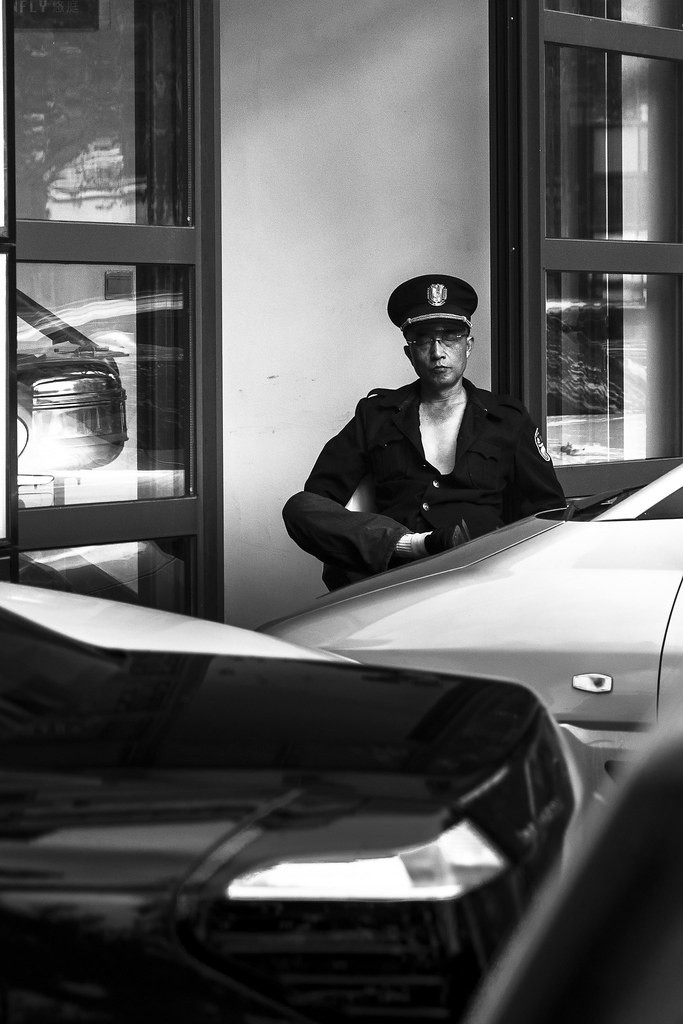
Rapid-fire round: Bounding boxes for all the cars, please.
[254,460,683,782]
[0,582,604,1024]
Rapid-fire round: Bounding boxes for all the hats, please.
[388,274,478,330]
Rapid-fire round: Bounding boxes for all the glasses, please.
[406,333,468,348]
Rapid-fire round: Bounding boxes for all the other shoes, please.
[425,513,473,556]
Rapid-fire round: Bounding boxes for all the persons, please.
[281,274,567,594]
